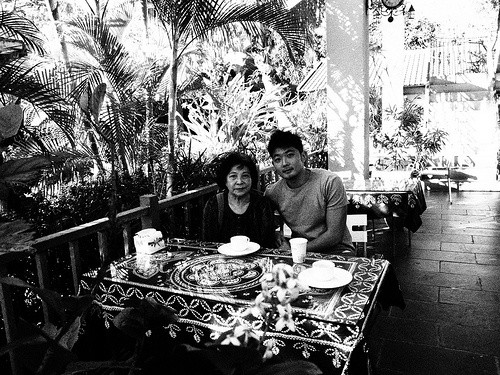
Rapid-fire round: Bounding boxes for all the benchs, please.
[274,213,367,256]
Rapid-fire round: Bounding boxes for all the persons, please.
[262,129,356,256]
[201,153,276,248]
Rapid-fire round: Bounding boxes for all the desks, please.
[74,238,406,375]
[333,172,427,255]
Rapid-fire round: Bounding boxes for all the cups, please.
[289,237,308,263]
[230,235,250,247]
[312,260,336,281]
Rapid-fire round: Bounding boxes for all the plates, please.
[297,268,353,288]
[217,241,261,257]
